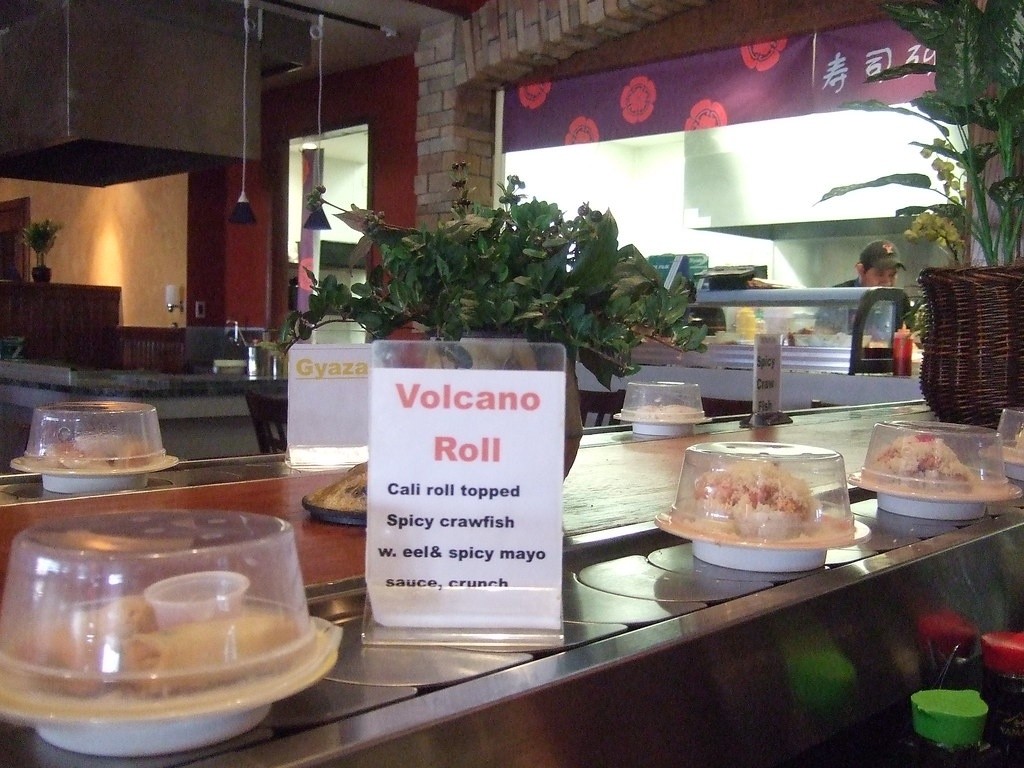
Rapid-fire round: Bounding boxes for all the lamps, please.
[163,283,183,314]
[303,13,330,228]
[230,0,256,225]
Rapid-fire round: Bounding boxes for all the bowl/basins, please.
[792,334,815,347]
[144,571,249,630]
[840,335,871,349]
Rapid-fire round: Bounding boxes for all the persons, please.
[813,239,925,350]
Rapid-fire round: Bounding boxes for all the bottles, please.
[243,346,261,380]
[270,356,283,380]
[893,321,913,377]
[736,307,755,340]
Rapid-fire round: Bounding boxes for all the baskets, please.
[919,265,1024,429]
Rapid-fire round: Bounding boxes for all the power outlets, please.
[195,301,206,318]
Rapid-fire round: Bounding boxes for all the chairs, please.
[243,390,287,454]
[580,387,626,424]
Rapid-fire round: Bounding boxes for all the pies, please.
[32,593,305,698]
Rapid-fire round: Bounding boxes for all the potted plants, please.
[20,218,64,282]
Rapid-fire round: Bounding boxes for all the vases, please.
[913,264,1024,427]
[429,332,582,490]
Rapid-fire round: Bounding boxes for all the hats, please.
[860,240,907,271]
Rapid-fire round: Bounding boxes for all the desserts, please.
[692,461,820,543]
[873,433,972,493]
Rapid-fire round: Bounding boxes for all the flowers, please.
[822,0,1024,271]
[263,154,712,369]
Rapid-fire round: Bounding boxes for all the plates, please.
[613,413,712,435]
[736,339,755,344]
[977,447,1024,482]
[0,622,338,756]
[10,456,180,494]
[654,507,872,573]
[847,471,1022,520]
